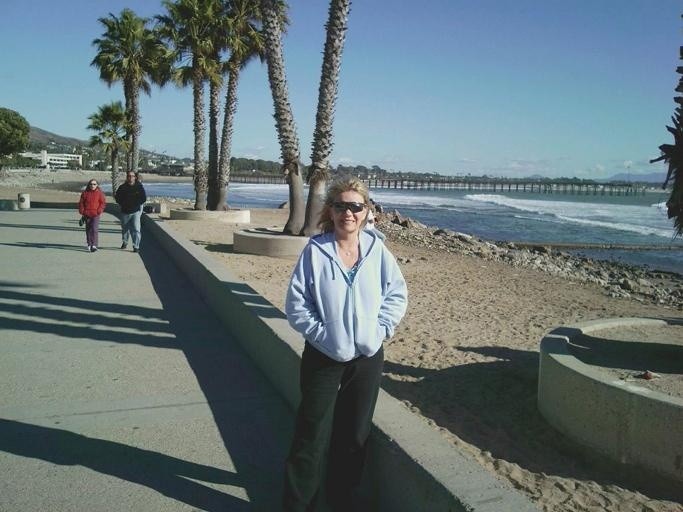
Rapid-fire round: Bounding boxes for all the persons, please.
[284,176,409,512]
[114,170,146,252]
[77,179,106,252]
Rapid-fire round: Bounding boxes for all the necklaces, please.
[336,239,359,256]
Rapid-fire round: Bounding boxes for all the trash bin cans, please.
[18,193,30,210]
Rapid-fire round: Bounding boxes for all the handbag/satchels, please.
[78,216,86,227]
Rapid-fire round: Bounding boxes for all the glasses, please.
[331,200,365,214]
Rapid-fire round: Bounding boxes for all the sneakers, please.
[86,243,138,252]
